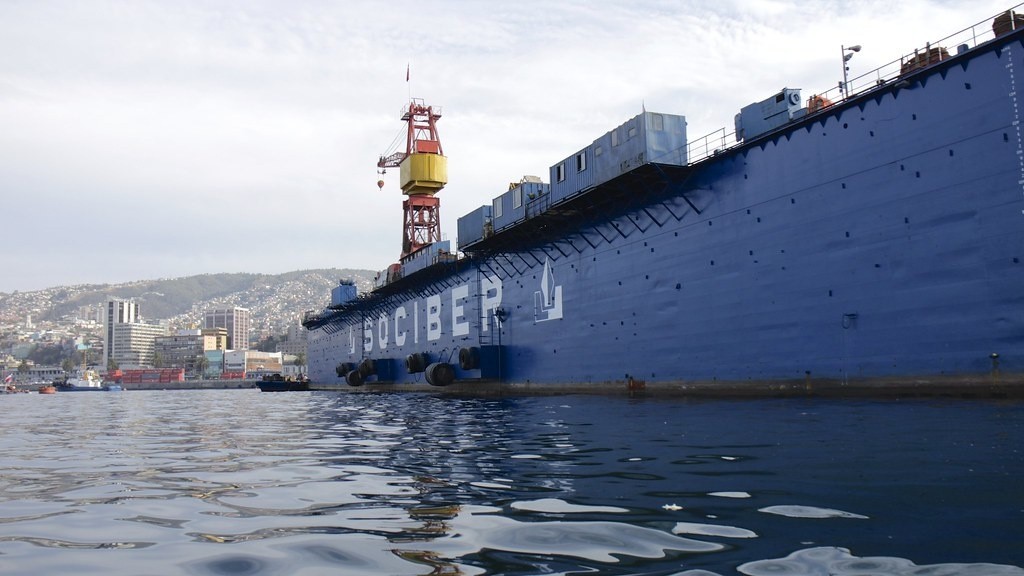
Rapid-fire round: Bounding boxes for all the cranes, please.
[376,96,448,260]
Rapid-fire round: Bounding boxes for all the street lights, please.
[841,45,862,97]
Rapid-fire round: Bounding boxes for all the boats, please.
[38,380,55,394]
[0,373,29,394]
[255,372,309,393]
[57,351,109,391]
